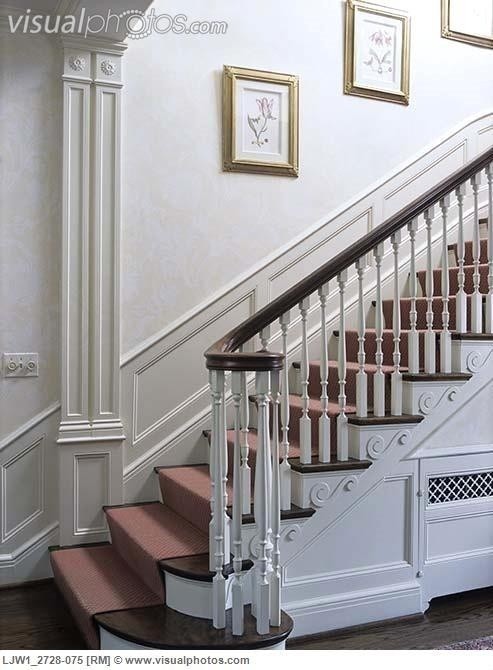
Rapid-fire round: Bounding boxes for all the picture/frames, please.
[344,0,410,107]
[222,65,299,179]
[441,1,492,50]
